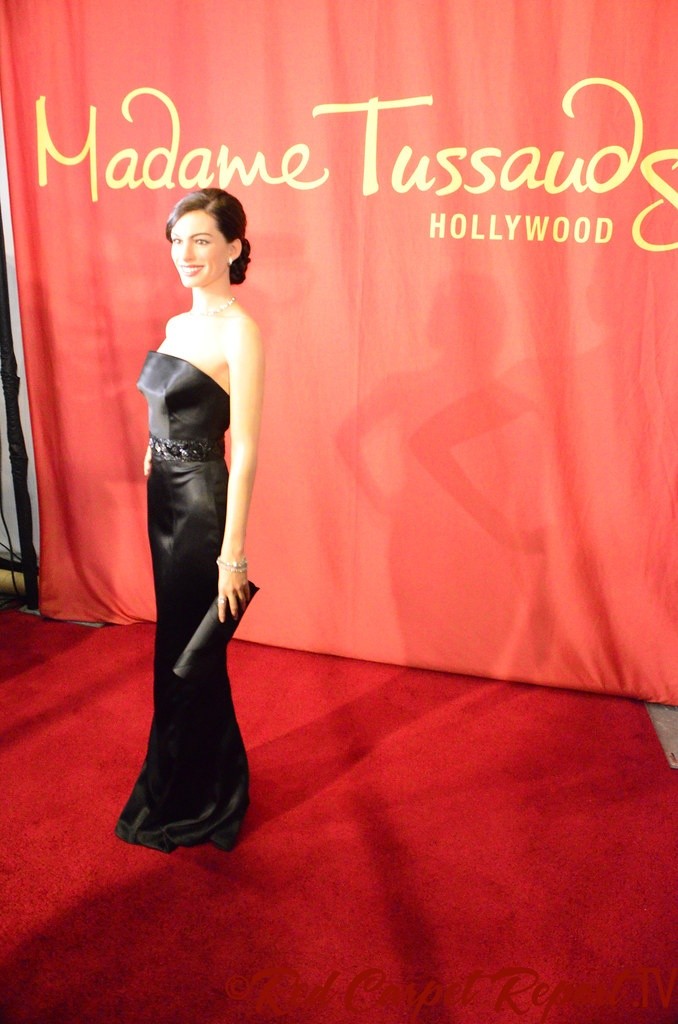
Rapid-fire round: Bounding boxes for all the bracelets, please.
[216,556,248,573]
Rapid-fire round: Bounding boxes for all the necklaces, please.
[193,296,236,316]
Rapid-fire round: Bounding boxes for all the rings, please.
[217,598,227,604]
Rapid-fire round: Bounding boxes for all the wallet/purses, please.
[174,579,261,684]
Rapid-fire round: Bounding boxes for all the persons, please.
[113,189,266,854]
